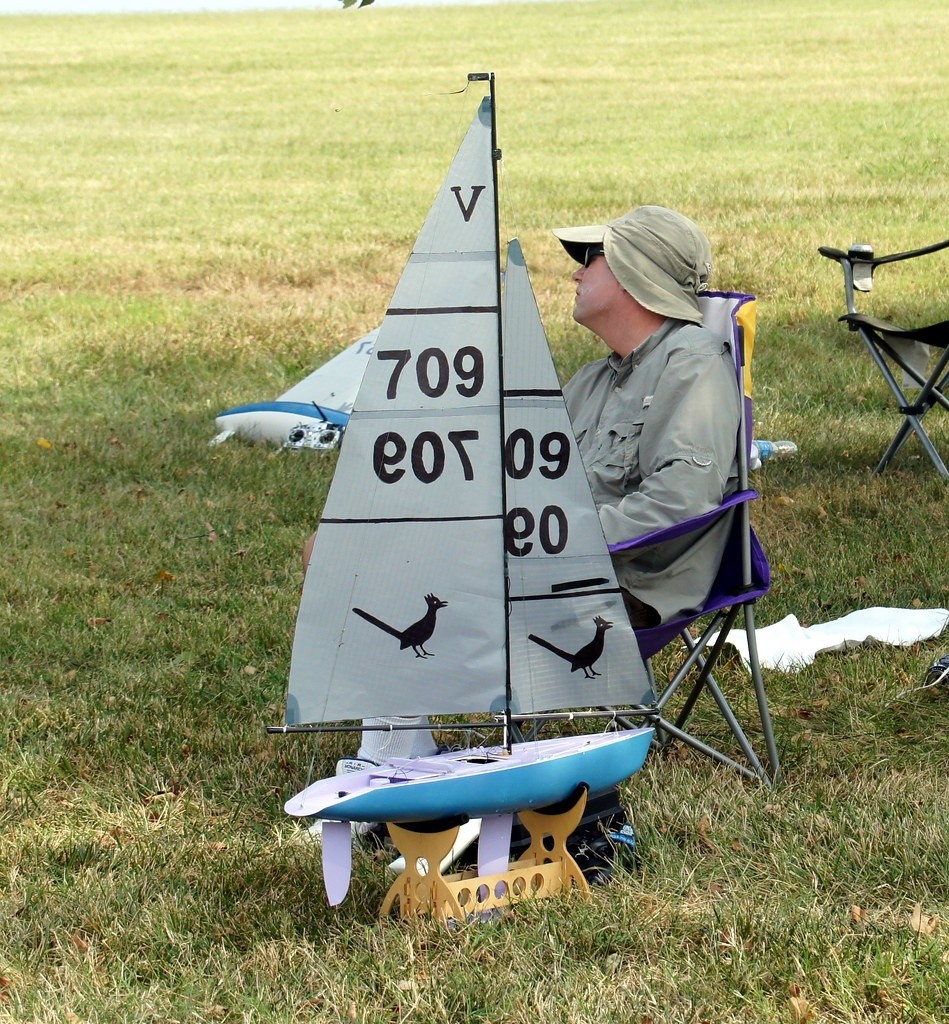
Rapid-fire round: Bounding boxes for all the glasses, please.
[583,244,605,270]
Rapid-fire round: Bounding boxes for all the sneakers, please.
[299,746,450,838]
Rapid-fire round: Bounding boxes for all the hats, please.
[554,204,714,328]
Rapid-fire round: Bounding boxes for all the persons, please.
[306,205,746,841]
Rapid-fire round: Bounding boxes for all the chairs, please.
[818,238,949,486]
[497,288,786,791]
